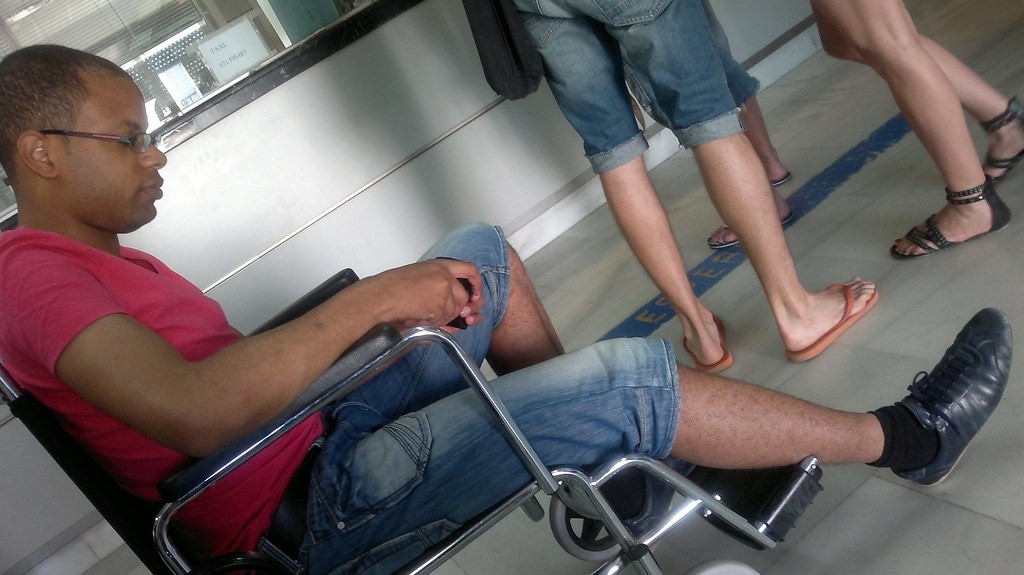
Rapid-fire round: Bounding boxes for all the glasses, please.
[37,128,164,155]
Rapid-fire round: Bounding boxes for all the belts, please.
[254,421,330,575]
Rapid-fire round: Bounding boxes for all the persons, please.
[690,1,797,251]
[0,42,1014,575]
[513,0,883,375]
[810,0,1022,262]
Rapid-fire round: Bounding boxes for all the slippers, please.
[681,312,733,376]
[708,209,794,249]
[771,171,791,186]
[784,283,879,362]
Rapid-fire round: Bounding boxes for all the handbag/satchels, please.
[460,0,545,102]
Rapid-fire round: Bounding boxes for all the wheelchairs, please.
[1,268,824,575]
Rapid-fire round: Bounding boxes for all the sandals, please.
[889,174,1011,259]
[982,95,1024,182]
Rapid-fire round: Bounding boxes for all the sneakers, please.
[668,454,698,476]
[896,308,1012,487]
